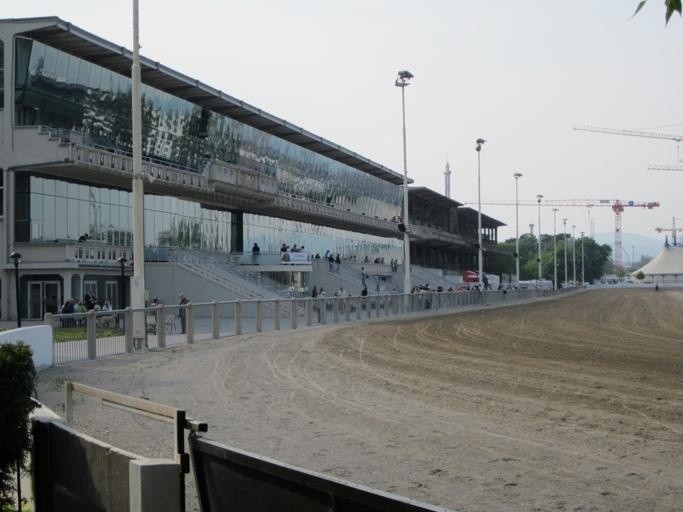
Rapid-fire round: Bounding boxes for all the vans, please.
[519,281,553,291]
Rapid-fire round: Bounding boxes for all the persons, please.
[498,281,502,290]
[656,283,659,290]
[61,288,114,327]
[252,243,260,265]
[178,293,190,334]
[281,243,480,313]
[151,299,159,321]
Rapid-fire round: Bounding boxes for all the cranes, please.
[464,198,660,277]
[571,123,683,172]
[657,218,682,245]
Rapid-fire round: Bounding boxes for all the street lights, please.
[394,70,413,313]
[552,208,586,288]
[116,257,127,308]
[513,171,522,287]
[535,194,544,281]
[9,252,23,328]
[476,138,486,294]
